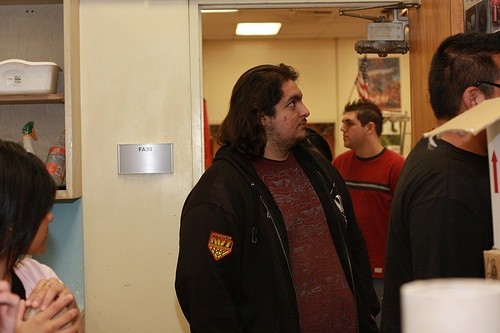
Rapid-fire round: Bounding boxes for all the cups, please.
[401,279,500,333]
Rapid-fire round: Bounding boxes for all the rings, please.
[41,281,55,289]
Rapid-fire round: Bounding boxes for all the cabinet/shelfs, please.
[0,0,81,200]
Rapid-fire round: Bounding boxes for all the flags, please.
[355,57,372,100]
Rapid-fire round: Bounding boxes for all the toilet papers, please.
[397,276,500,333]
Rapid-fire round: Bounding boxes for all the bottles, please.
[45,129,65,187]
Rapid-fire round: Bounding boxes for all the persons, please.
[332,100,406,302]
[0,139,83,333]
[175,63,381,333]
[381,34,500,333]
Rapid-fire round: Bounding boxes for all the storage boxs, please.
[0,59,63,95]
